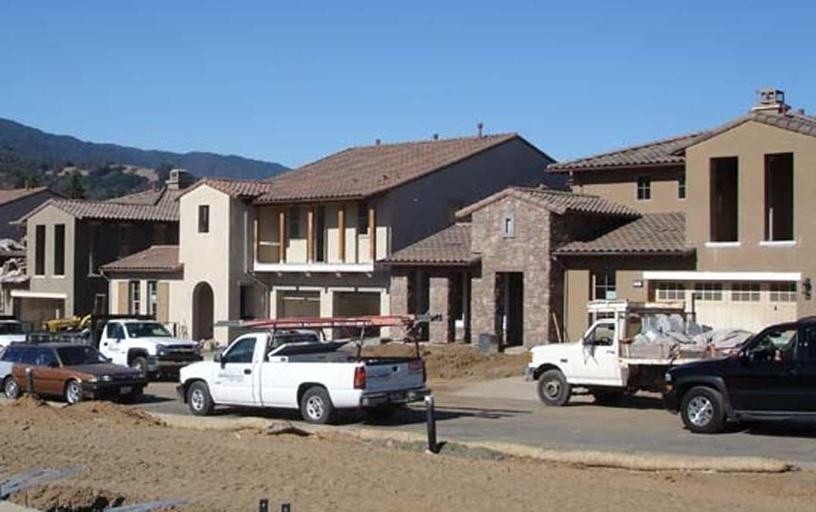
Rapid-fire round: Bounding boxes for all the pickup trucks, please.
[524,297,816,434]
